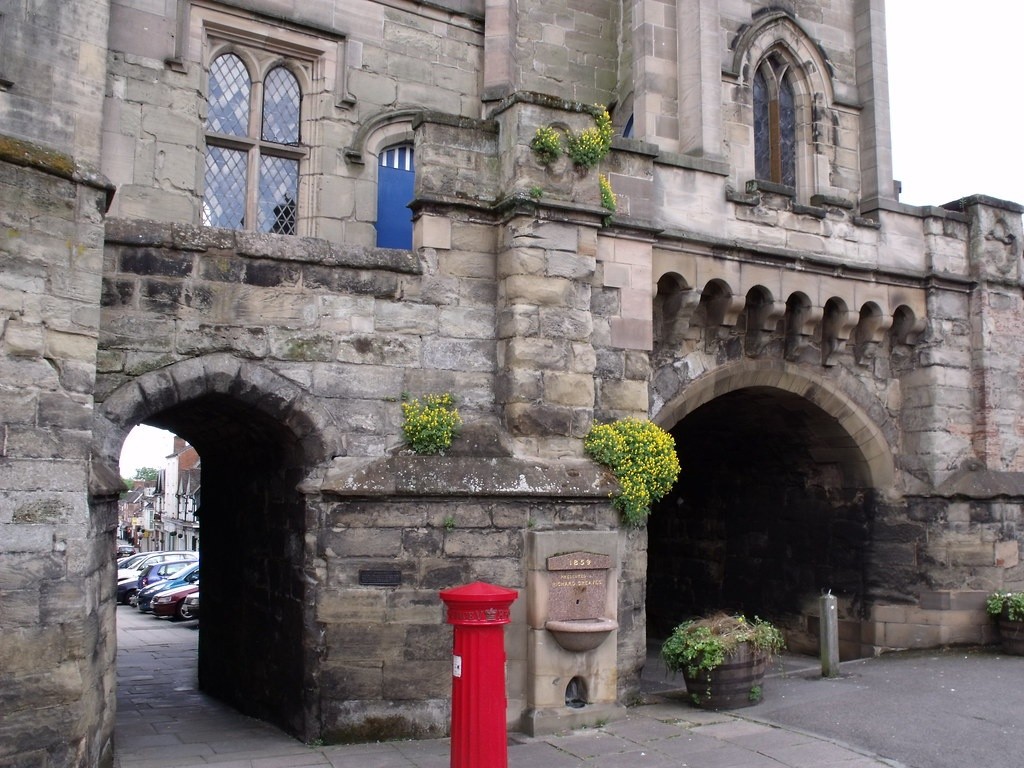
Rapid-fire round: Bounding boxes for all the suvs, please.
[117,551,199,581]
[137,561,199,597]
[117,545,136,558]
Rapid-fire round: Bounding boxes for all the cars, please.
[117,557,128,563]
[182,592,199,620]
[117,576,138,607]
[150,578,199,619]
[117,552,165,571]
[137,561,199,612]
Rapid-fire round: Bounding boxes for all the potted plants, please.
[985,589,1024,657]
[658,612,787,712]
